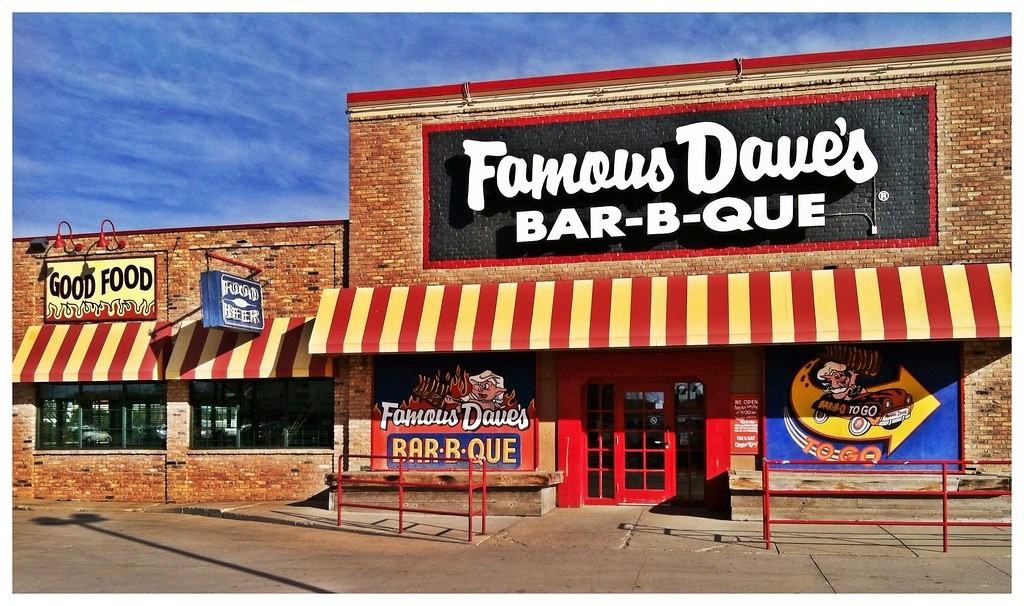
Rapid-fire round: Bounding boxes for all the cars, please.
[73,426,112,443]
